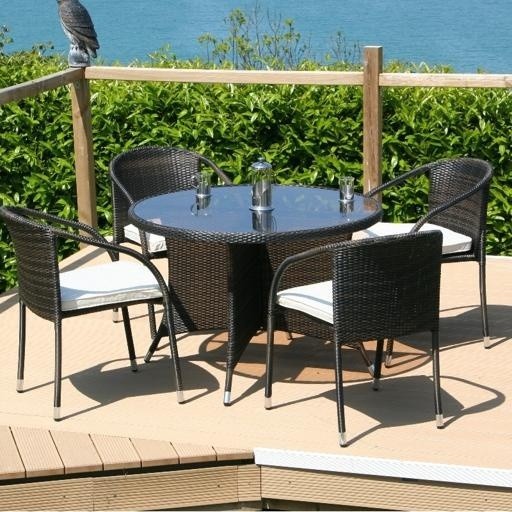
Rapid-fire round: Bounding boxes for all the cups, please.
[338,177,355,201]
[190,172,212,195]
[338,201,355,217]
[190,195,212,216]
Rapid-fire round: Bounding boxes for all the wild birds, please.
[57,0,100,60]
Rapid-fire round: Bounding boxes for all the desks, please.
[128,184,383,406]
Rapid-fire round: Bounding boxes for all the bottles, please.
[249,157,275,210]
[249,210,278,233]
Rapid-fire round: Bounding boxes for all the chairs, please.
[1,204,185,421]
[350,158,493,368]
[263,230,445,447]
[108,146,232,339]
